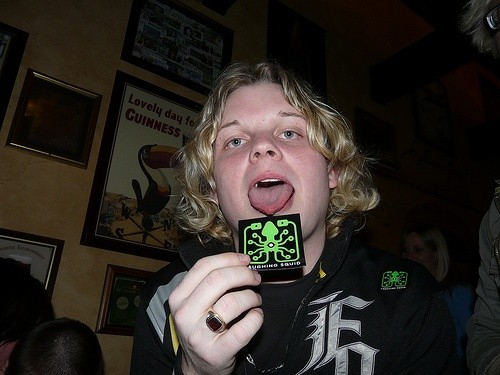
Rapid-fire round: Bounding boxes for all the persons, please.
[0,259,105,375]
[128,58,458,375]
[400,221,475,357]
[466,189,500,375]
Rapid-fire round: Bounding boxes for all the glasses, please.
[483,3,500,34]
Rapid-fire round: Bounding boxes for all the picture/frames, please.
[5,67,103,168]
[95,264,156,336]
[0,228,65,302]
[120,0,234,95]
[81,70,203,261]
[0,21,30,130]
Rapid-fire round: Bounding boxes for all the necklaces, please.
[242,349,285,373]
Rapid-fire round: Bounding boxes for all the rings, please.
[206,309,226,332]
